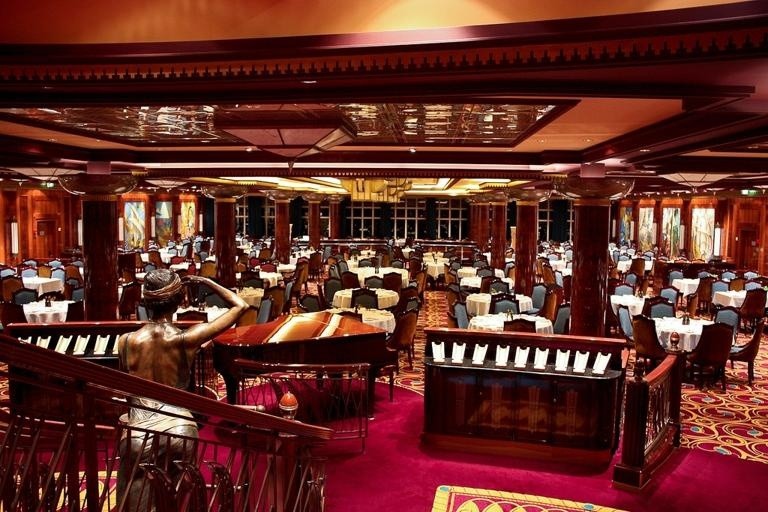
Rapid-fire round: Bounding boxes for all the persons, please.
[115,267,250,511]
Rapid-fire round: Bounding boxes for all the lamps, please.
[6,163,84,183]
[656,171,732,188]
[214,117,356,159]
[145,177,186,189]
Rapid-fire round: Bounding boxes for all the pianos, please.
[211,311,398,419]
